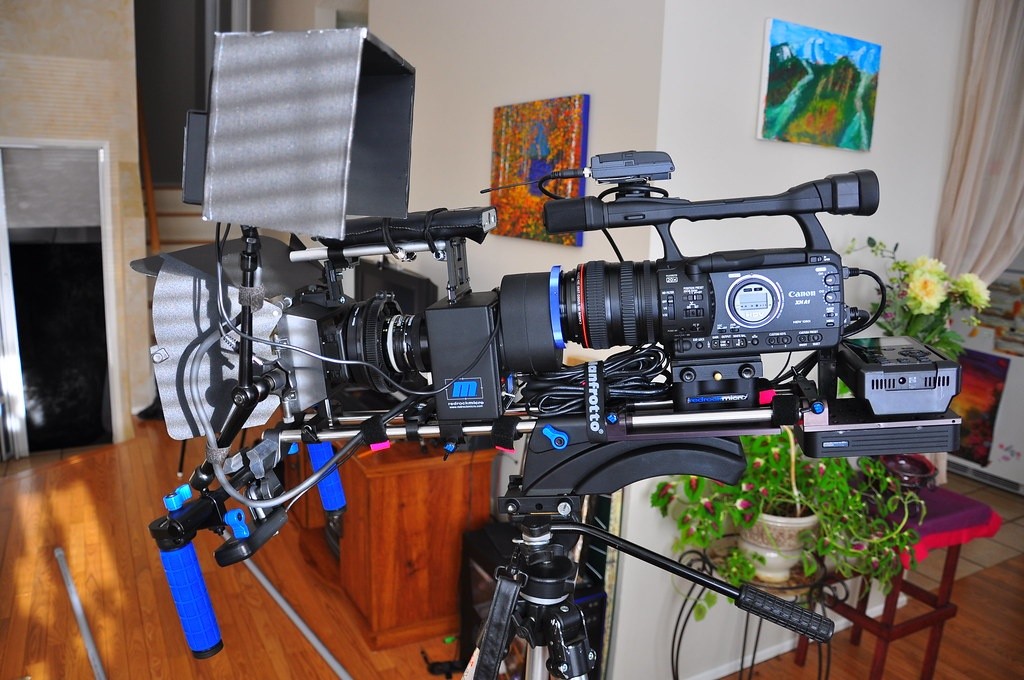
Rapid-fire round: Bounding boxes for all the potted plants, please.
[652,428,929,601]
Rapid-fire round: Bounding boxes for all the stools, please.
[796,478,1003,680]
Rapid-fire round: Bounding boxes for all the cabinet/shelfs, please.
[285,438,498,652]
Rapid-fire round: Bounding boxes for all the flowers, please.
[845,236,993,364]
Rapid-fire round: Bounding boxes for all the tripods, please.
[460,514,835,680]
